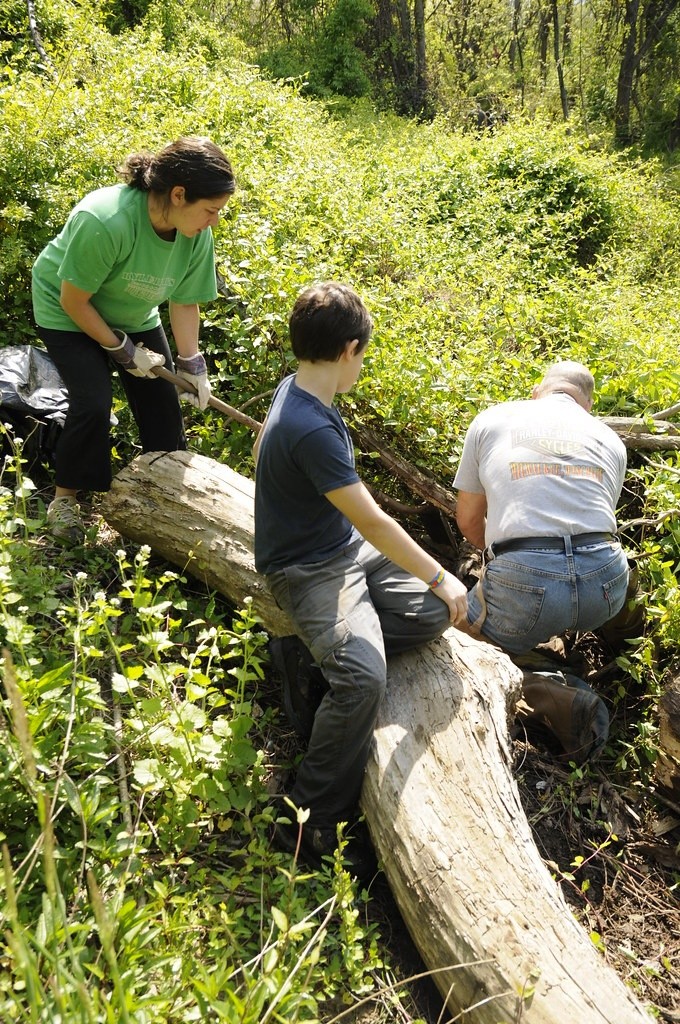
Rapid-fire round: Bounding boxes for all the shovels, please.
[149,364,456,550]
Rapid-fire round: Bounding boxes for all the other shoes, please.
[270,805,378,887]
[522,674,609,766]
[268,634,321,721]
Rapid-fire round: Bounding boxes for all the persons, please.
[250,280,472,869]
[31,136,236,548]
[450,360,631,659]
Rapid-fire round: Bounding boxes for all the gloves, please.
[173,353,213,412]
[99,328,166,380]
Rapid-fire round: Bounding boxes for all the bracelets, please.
[426,566,448,592]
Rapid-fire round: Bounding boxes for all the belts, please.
[482,530,619,561]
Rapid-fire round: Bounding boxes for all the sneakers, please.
[45,495,86,541]
